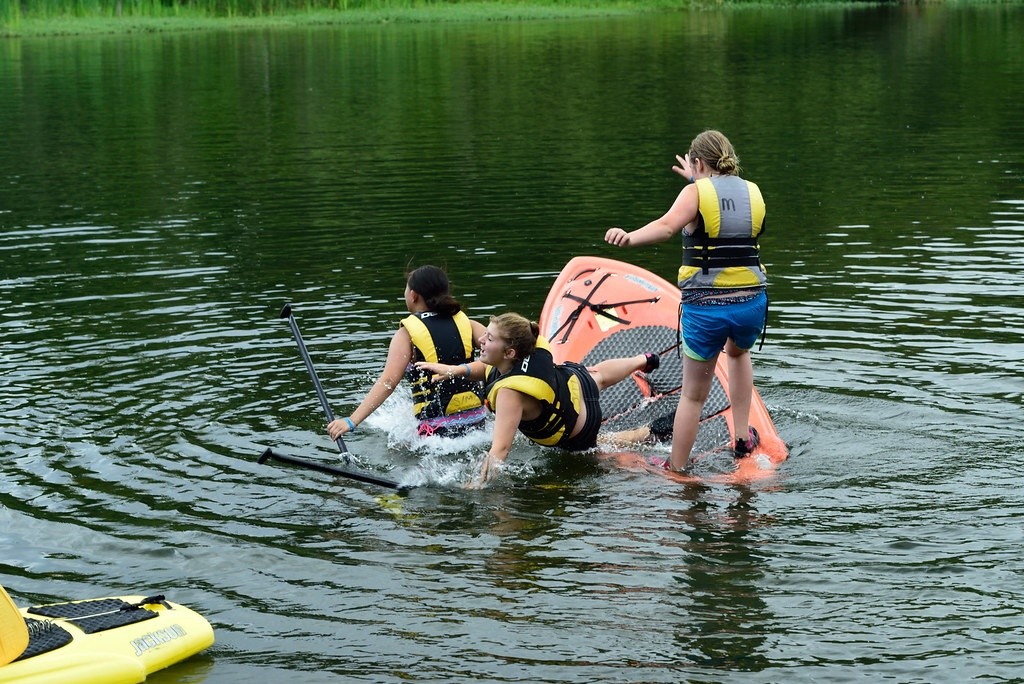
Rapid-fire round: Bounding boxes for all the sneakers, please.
[643,351,660,374]
[728,425,762,458]
[649,455,697,477]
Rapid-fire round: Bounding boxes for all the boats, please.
[538,251,789,490]
[2,592,217,684]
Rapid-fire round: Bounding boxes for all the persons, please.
[605,130,768,475]
[413,313,660,488]
[326,265,488,442]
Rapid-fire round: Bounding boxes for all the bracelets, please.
[691,175,695,182]
[345,416,356,432]
[460,363,471,379]
